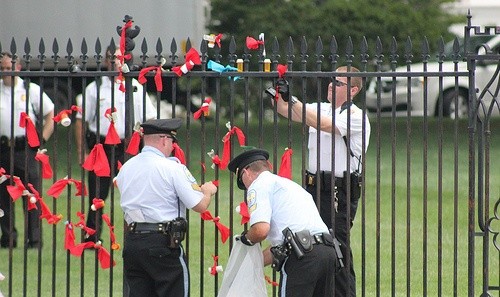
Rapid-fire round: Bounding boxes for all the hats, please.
[228,149,269,190]
[140,119,182,144]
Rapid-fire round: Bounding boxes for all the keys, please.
[335,201,338,213]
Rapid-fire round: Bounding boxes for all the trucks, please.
[0,0,212,124]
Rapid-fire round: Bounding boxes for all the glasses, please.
[331,78,354,87]
[160,135,176,144]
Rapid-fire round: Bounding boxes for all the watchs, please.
[292,95,297,105]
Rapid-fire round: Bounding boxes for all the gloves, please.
[275,77,289,102]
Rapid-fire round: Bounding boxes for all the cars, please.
[365,21,500,118]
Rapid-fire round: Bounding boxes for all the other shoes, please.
[26,240,41,249]
[0,236,17,248]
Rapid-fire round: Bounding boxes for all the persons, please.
[227,148,336,297]
[75,43,156,249]
[265,65,371,297]
[116,119,217,297]
[0,51,60,248]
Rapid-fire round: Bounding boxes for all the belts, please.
[130,223,165,231]
[311,236,324,246]
[306,174,343,188]
